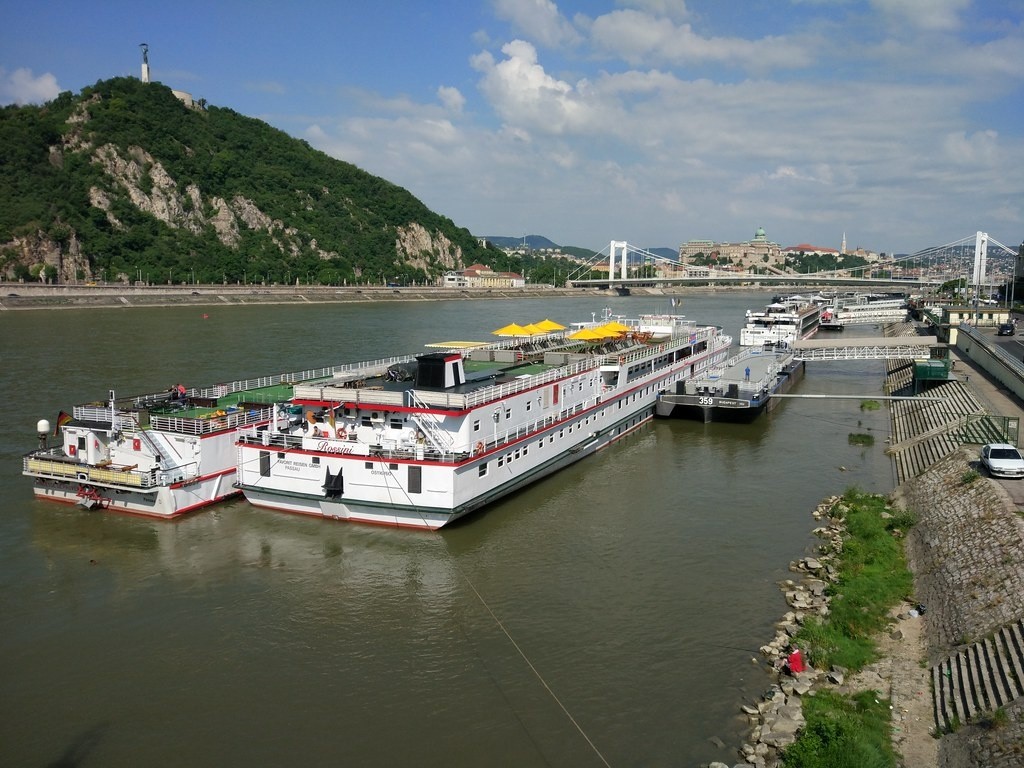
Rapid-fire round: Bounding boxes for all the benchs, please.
[517,351,529,361]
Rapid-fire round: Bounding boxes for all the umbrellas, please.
[536,319,567,341]
[492,323,534,350]
[591,326,624,351]
[567,328,605,354]
[521,323,550,344]
[605,322,633,345]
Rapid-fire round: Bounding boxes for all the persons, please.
[745,366,750,381]
[313,426,322,437]
[169,384,177,400]
[176,384,186,405]
[779,645,804,675]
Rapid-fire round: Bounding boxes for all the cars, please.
[998,323,1015,336]
[981,444,1024,479]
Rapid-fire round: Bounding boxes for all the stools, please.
[494,374,532,385]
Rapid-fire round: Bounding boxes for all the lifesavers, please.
[660,344,665,352]
[416,429,426,439]
[620,355,624,366]
[336,427,348,440]
[475,442,483,455]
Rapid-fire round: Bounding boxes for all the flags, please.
[53,411,73,437]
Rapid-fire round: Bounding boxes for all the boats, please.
[232,294,732,530]
[740,290,888,345]
[21,341,493,519]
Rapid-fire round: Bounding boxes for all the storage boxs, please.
[494,350,519,363]
[544,352,570,367]
[471,350,493,361]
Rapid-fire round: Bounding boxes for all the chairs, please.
[142,397,188,416]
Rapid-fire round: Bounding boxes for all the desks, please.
[237,402,269,411]
[188,397,217,406]
